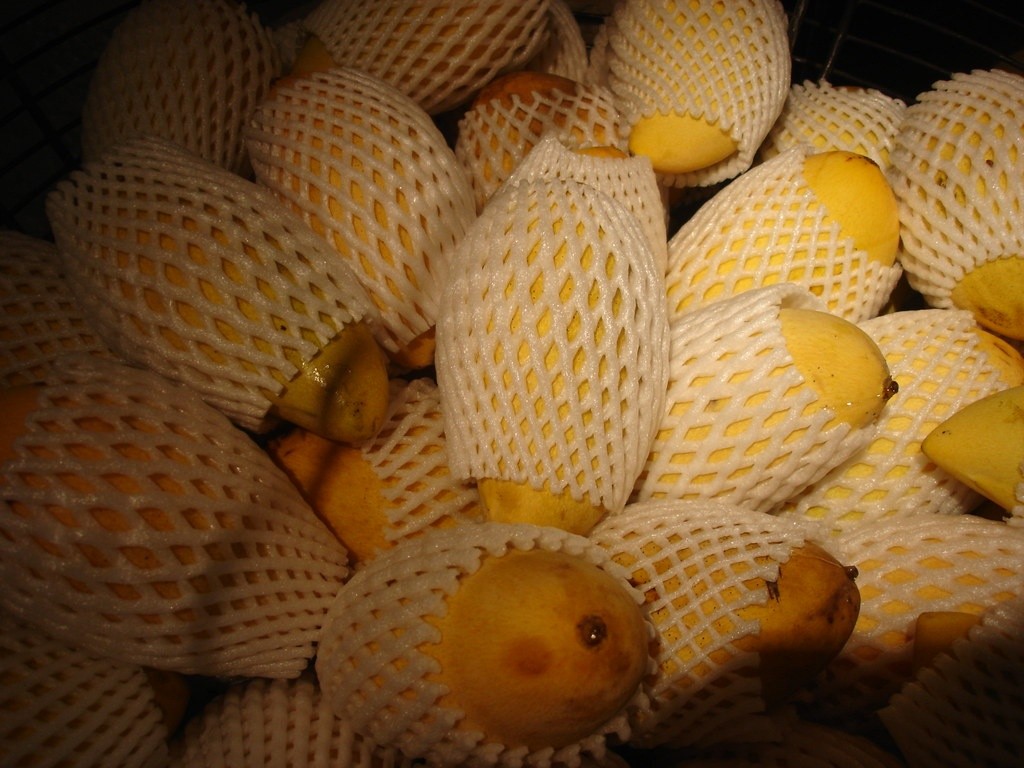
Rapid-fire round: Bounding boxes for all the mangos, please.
[0,0,1024,768]
